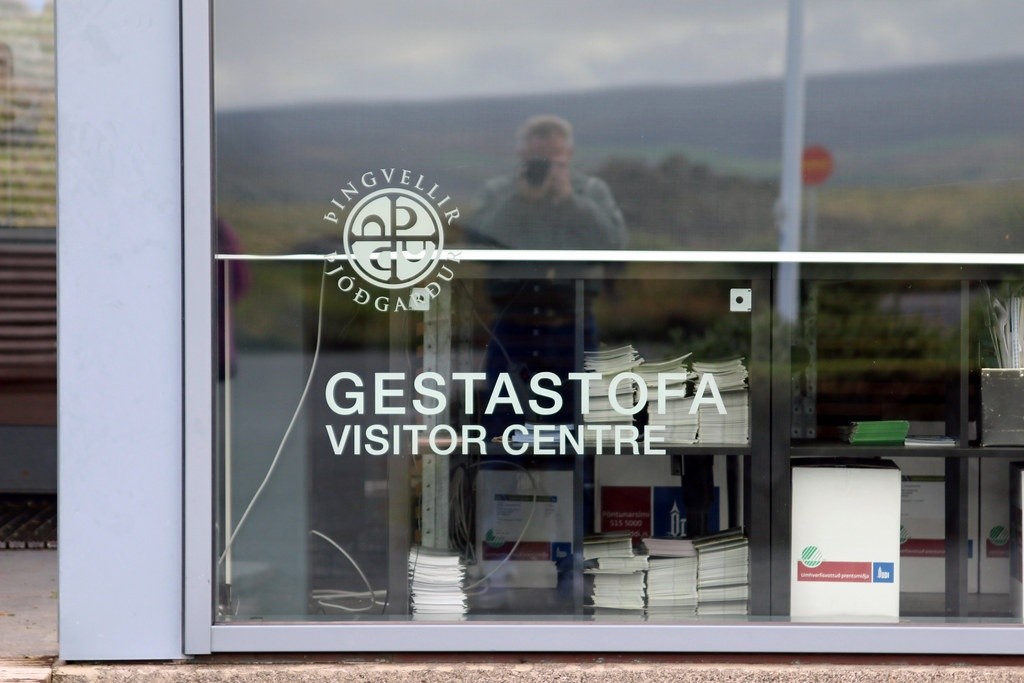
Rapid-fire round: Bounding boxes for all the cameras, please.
[521,156,562,188]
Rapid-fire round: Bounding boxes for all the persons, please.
[465,112,624,606]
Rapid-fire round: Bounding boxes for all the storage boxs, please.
[593,454,687,539]
[474,470,572,586]
[790,460,901,623]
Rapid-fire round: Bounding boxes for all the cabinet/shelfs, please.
[388,261,1023,622]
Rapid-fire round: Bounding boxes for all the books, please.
[976,280,1024,369]
[579,346,750,445]
[582,526,750,621]
[834,419,956,447]
[408,543,471,621]
